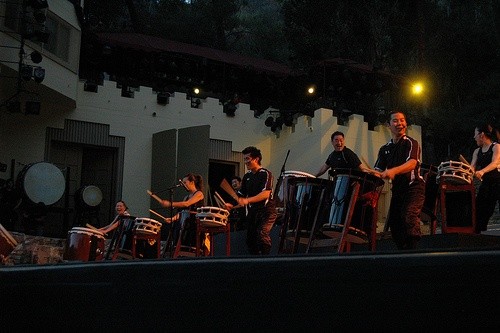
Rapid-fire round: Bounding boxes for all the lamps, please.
[23,66,46,82]
[157,92,168,105]
[33,10,46,24]
[264,112,294,133]
[35,30,49,44]
[224,102,237,117]
[8,100,41,115]
[31,50,42,64]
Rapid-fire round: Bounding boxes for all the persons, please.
[98,201,131,236]
[232,176,241,193]
[374,112,425,238]
[470,122,500,231]
[225,146,277,249]
[163,174,204,223]
[314,131,369,178]
[0,180,24,227]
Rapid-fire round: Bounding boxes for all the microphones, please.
[178,179,190,192]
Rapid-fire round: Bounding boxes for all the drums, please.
[285,172,327,244]
[439,160,471,186]
[69,227,106,260]
[134,217,162,234]
[331,172,385,243]
[283,171,316,179]
[197,206,229,226]
